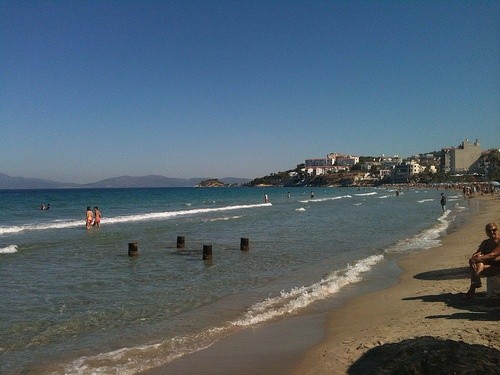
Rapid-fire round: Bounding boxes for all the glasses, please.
[487,229,497,232]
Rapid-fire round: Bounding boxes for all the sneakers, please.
[462,290,475,297]
[471,279,481,288]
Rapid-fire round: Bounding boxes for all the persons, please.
[440,193,448,214]
[308,191,314,198]
[82,206,103,230]
[382,181,495,197]
[40,202,51,210]
[264,193,270,203]
[287,192,291,198]
[460,223,500,298]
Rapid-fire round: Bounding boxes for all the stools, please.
[486,276,500,297]
